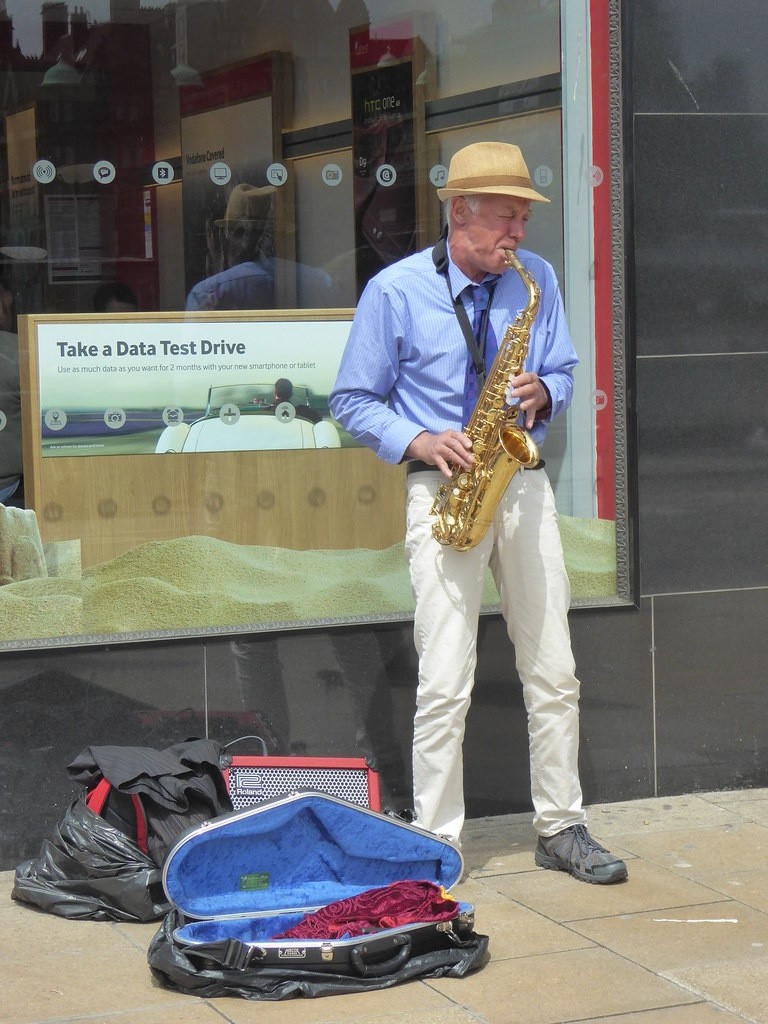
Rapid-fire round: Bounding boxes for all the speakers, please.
[217,753,381,814]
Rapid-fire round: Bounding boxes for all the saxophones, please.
[426,245,542,554]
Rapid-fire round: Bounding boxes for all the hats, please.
[437,142,551,202]
[215,184,292,230]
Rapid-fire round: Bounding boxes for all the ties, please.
[462,275,502,433]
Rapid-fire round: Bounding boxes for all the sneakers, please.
[534,824,629,885]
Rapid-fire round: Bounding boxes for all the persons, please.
[0,279,25,509]
[261,378,323,423]
[327,142,628,885]
[184,182,343,310]
[93,283,139,313]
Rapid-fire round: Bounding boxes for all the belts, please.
[406,460,545,472]
